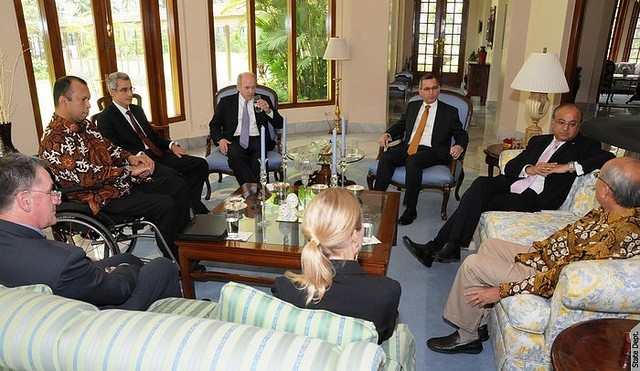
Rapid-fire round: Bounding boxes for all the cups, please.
[224,211,240,237]
[363,213,375,243]
[253,95,263,113]
[229,198,243,206]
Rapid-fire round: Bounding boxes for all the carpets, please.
[122,157,495,370]
[599,94,640,107]
[581,115,640,153]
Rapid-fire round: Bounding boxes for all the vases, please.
[0,122,20,159]
[623,66,630,77]
[479,46,487,64]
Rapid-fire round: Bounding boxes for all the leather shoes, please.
[478,324,489,342]
[431,242,461,263]
[397,209,418,226]
[402,236,439,268]
[426,331,483,354]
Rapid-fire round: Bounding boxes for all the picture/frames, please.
[486,6,496,47]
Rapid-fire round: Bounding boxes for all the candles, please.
[330,128,339,188]
[339,116,346,187]
[280,116,287,205]
[258,125,269,225]
[283,234,288,252]
[259,225,269,243]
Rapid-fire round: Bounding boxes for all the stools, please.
[395,71,413,91]
[389,81,408,106]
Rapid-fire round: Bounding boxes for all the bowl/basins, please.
[274,194,286,204]
[311,184,328,194]
[275,182,290,194]
[346,184,364,197]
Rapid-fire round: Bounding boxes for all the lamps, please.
[511,48,570,149]
[323,36,351,134]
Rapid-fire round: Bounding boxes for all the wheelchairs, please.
[34,154,182,280]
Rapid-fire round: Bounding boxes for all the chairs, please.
[97,94,169,141]
[206,85,283,200]
[367,90,473,221]
[601,60,615,104]
[90,110,103,129]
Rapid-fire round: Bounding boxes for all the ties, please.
[407,105,431,156]
[239,100,250,149]
[126,110,165,159]
[511,141,560,194]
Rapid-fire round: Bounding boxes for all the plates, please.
[224,202,247,210]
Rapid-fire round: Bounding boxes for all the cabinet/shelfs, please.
[467,64,490,106]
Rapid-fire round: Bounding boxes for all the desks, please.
[613,77,640,104]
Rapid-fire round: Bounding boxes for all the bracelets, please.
[568,161,574,173]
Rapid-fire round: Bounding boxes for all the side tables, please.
[484,143,524,177]
[551,318,640,371]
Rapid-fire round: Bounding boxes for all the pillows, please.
[614,63,619,74]
[620,63,636,74]
[0,284,52,293]
[218,282,379,346]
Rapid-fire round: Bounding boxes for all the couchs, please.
[613,63,640,90]
[473,149,640,371]
[0,289,415,371]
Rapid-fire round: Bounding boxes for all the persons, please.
[38,75,208,282]
[97,72,210,215]
[427,156,640,355]
[209,72,283,186]
[403,103,616,268]
[374,74,469,225]
[271,187,402,345]
[0,151,210,310]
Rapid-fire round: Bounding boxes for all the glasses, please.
[117,86,135,94]
[593,170,615,194]
[554,118,581,128]
[420,86,439,91]
[31,190,63,206]
